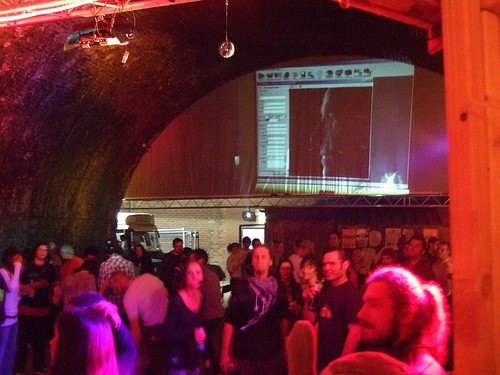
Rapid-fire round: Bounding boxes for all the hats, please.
[58,244,73,253]
[104,239,122,253]
[82,245,100,257]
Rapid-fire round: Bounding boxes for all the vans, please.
[115,212,167,271]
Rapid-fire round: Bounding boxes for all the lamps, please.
[62,0,136,50]
[219,0,235,58]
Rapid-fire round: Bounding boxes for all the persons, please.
[304,247,362,361]
[65,292,105,313]
[0,252,24,375]
[356,267,452,374]
[48,302,136,374]
[54,259,99,302]
[19,242,55,373]
[317,352,410,375]
[165,260,214,375]
[107,271,171,375]
[218,245,290,375]
[0,195,449,308]
